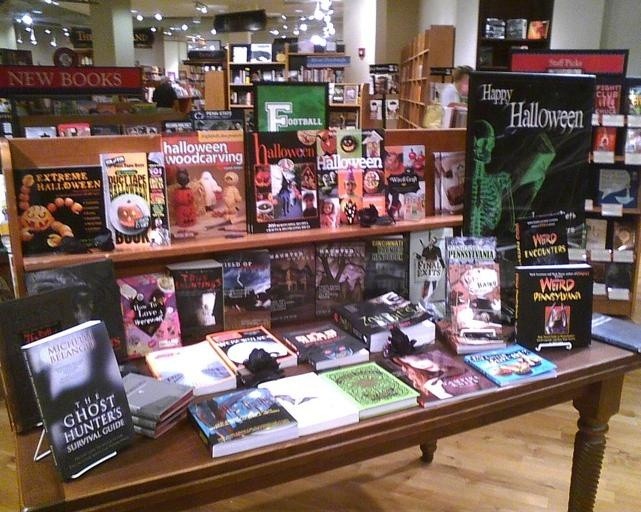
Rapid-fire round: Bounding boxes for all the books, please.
[1,43,641,485]
[507,19,527,39]
[484,17,505,39]
[526,20,551,39]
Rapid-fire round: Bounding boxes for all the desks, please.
[0,304,640,511]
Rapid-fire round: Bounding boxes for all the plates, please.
[108,193,151,235]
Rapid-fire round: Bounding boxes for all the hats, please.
[344,165,355,183]
